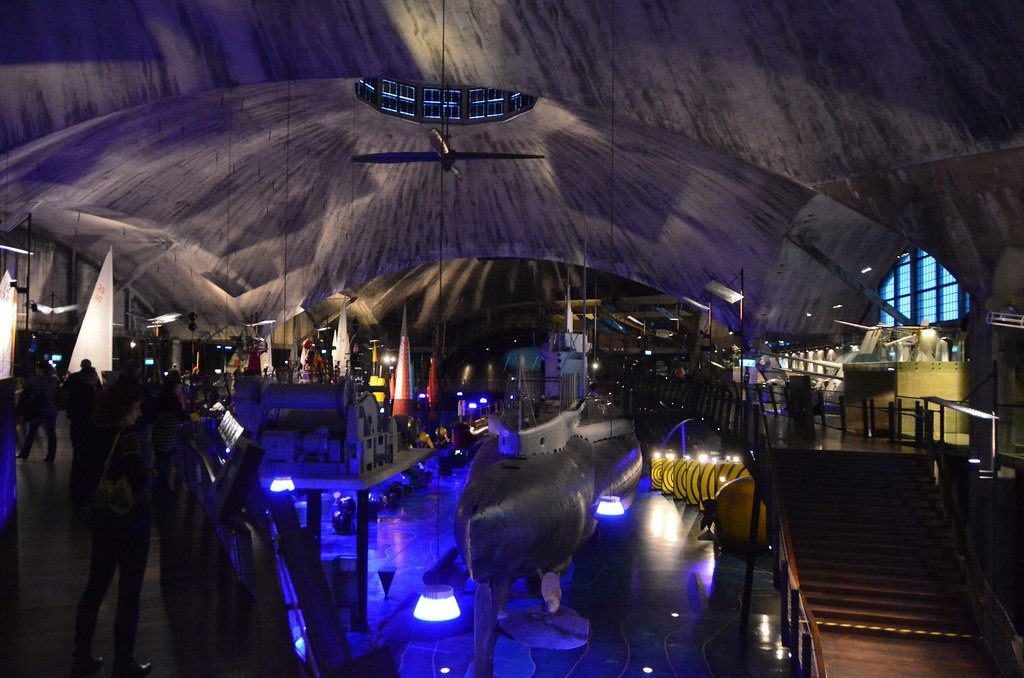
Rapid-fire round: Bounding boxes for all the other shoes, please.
[16,452,28,460]
[112,662,151,678]
[70,656,104,678]
[44,455,55,462]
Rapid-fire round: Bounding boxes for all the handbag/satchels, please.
[95,429,133,514]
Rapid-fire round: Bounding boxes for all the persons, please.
[70,380,159,678]
[15,359,200,516]
[675,364,685,380]
[292,360,303,381]
[233,366,247,389]
